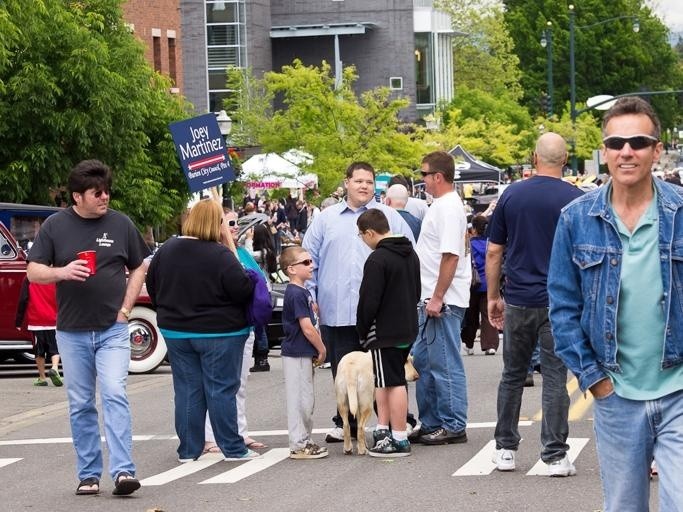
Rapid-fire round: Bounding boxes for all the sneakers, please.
[484,348,495,355]
[524,374,534,387]
[324,426,344,443]
[368,432,411,457]
[490,447,516,470]
[548,455,576,476]
[48,368,62,386]
[461,344,473,355]
[308,441,328,451]
[373,428,390,446]
[289,444,327,458]
[32,378,48,387]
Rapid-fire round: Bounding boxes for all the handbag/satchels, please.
[469,267,480,290]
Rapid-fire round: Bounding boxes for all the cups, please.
[78,251,96,276]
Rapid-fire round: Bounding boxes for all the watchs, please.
[120,307,130,318]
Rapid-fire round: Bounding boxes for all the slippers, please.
[224,449,263,461]
[76,477,99,495]
[203,445,221,453]
[244,440,268,449]
[111,472,140,495]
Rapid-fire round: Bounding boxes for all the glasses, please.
[226,219,240,227]
[284,258,311,267]
[603,134,658,150]
[86,189,108,197]
[357,232,368,238]
[420,170,437,176]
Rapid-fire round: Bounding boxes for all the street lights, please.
[566,4,644,124]
[216,110,231,209]
[541,21,553,122]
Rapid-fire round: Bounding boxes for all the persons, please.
[356,208,421,459]
[146,197,263,462]
[652,160,681,186]
[650,455,658,480]
[203,207,268,452]
[408,150,472,445]
[25,158,152,495]
[302,162,416,444]
[547,97,682,510]
[486,132,586,477]
[374,175,433,381]
[460,198,503,355]
[237,186,348,372]
[523,336,541,387]
[15,274,64,387]
[280,245,329,459]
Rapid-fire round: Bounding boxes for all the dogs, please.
[335,350,420,455]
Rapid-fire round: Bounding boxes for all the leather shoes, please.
[419,428,467,444]
[407,427,442,443]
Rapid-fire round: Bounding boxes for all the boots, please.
[249,350,270,372]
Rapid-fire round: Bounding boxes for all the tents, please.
[411,143,506,200]
[235,151,319,189]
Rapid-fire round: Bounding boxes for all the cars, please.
[0,202,63,254]
[0,221,168,374]
